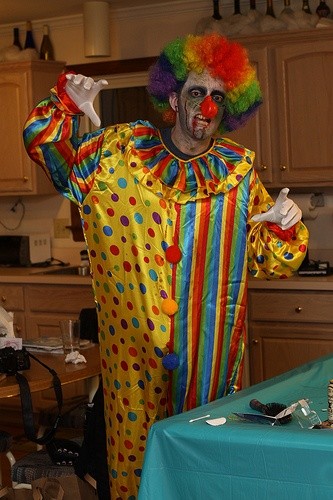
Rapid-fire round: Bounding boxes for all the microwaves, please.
[0,232,52,266]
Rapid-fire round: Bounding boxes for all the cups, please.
[60,319,81,355]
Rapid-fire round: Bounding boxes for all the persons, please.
[23,29,310,500]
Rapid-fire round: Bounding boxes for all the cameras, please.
[0,347,31,373]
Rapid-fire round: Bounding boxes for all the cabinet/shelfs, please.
[0,60,66,195]
[0,282,333,421]
[217,30,332,193]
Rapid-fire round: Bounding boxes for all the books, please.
[22,335,96,354]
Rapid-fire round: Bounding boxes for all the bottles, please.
[232,0,242,15]
[249,0,256,10]
[39,25,55,61]
[302,0,312,14]
[281,0,294,15]
[24,21,36,51]
[316,0,330,17]
[211,0,223,20]
[13,28,23,51]
[266,0,276,19]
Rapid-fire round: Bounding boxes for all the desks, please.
[135,355,333,500]
[0,337,100,399]
